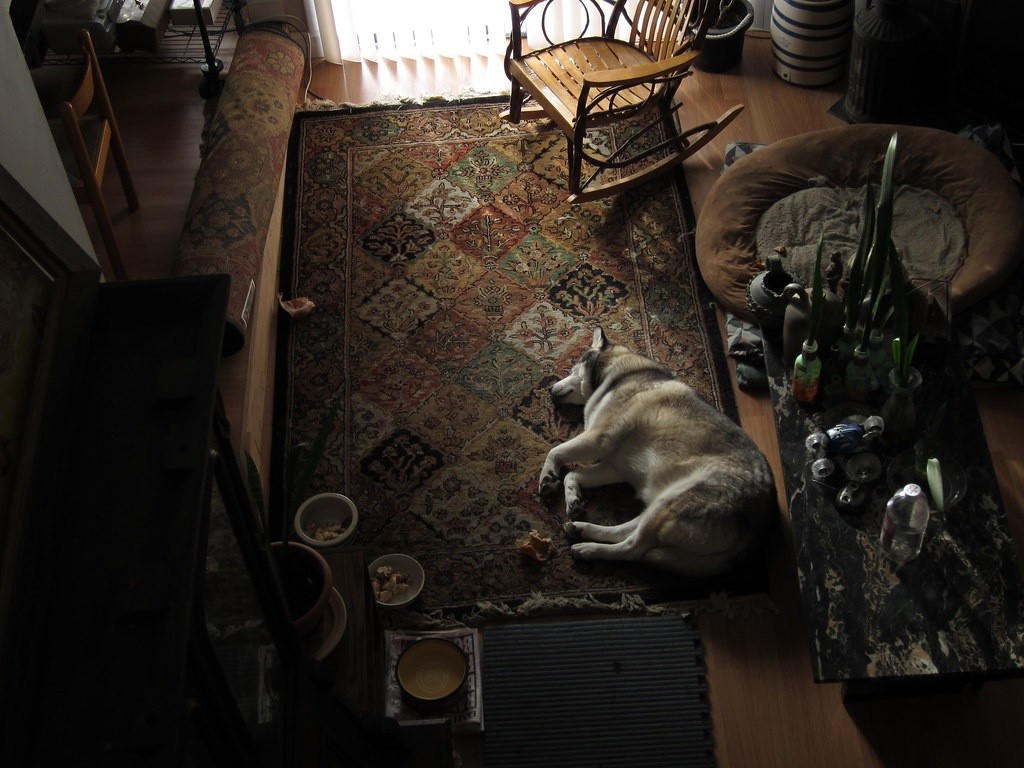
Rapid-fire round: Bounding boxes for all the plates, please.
[884,452,969,514]
[300,588,349,663]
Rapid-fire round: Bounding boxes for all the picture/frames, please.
[0,164,106,665]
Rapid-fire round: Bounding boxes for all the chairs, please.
[28,29,141,281]
[694,121,1024,331]
[502,1,745,205]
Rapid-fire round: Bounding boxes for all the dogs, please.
[535,323,781,580]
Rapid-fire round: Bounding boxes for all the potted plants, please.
[237,394,342,644]
[791,129,924,450]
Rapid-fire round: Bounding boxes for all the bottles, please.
[784,288,843,366]
[879,484,930,562]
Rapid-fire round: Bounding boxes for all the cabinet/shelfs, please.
[0,271,319,767]
[11,0,249,98]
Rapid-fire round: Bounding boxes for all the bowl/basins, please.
[294,494,360,548]
[368,554,425,609]
[269,541,333,631]
[394,637,468,700]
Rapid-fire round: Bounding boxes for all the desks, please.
[758,317,1024,708]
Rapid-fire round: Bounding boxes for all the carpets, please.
[168,28,307,359]
[373,615,720,768]
[269,98,779,627]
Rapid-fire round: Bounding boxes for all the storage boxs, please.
[115,0,172,53]
[168,0,223,26]
